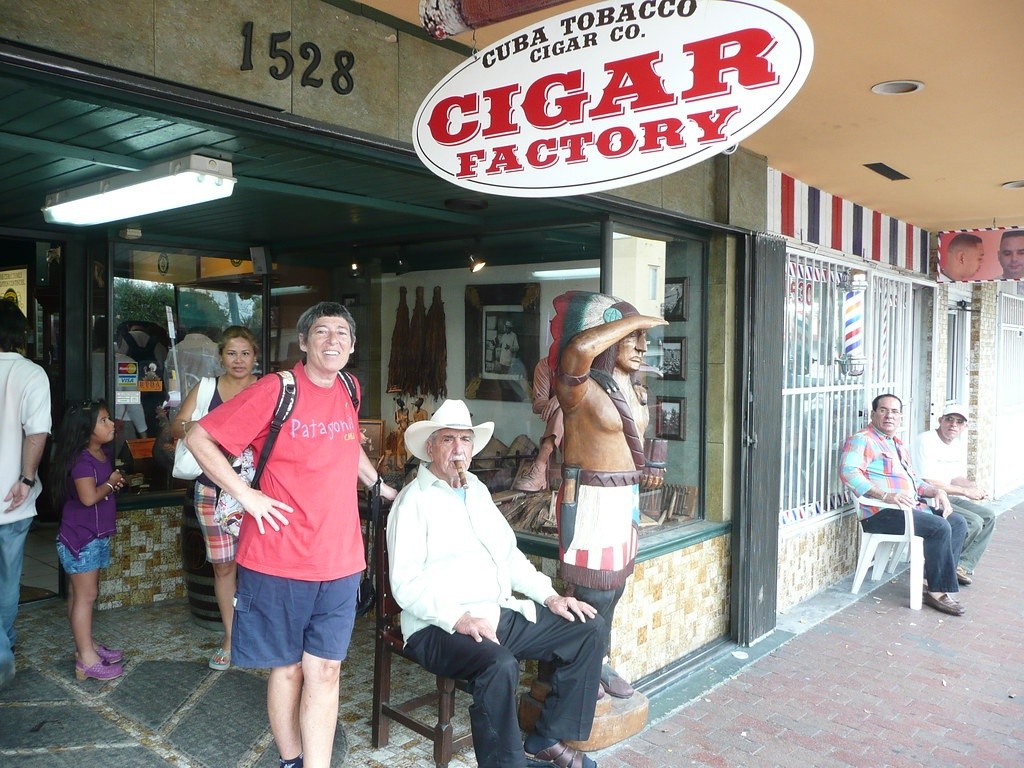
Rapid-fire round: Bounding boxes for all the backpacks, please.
[216,370,359,536]
[124,332,167,417]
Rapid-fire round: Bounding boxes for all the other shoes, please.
[209,644,232,671]
[955,566,973,585]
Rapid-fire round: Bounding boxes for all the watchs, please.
[18,474,37,488]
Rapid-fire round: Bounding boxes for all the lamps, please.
[41,154,238,227]
[842,269,868,376]
[463,235,486,274]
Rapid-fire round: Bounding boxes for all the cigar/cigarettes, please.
[453,460,468,489]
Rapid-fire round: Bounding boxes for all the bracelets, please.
[882,492,887,502]
[104,483,114,494]
[181,420,188,435]
[368,476,384,493]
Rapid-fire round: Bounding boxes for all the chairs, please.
[850,490,924,610]
[372,509,521,768]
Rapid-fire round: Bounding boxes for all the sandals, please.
[76,645,124,664]
[76,658,123,681]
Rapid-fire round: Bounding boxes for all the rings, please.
[899,498,902,501]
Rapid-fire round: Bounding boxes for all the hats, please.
[404,399,495,462]
[943,400,969,421]
[506,322,511,325]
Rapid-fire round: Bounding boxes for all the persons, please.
[993,230,1024,279]
[168,325,263,670]
[910,404,996,584]
[940,234,985,282]
[0,297,52,689]
[494,321,520,373]
[68,305,227,492]
[184,301,398,768]
[55,398,123,681]
[513,290,669,696]
[661,287,681,434]
[386,399,601,768]
[838,394,968,615]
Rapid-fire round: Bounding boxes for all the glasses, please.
[942,416,965,424]
[506,327,511,329]
[874,408,902,416]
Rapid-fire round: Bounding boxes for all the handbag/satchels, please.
[356,475,381,617]
[170,377,218,479]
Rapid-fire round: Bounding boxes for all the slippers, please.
[523,740,597,768]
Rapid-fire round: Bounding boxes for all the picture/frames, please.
[465,283,540,403]
[655,396,686,441]
[657,337,688,381]
[665,277,689,321]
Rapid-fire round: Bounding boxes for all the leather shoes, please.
[924,588,964,616]
[515,461,547,491]
